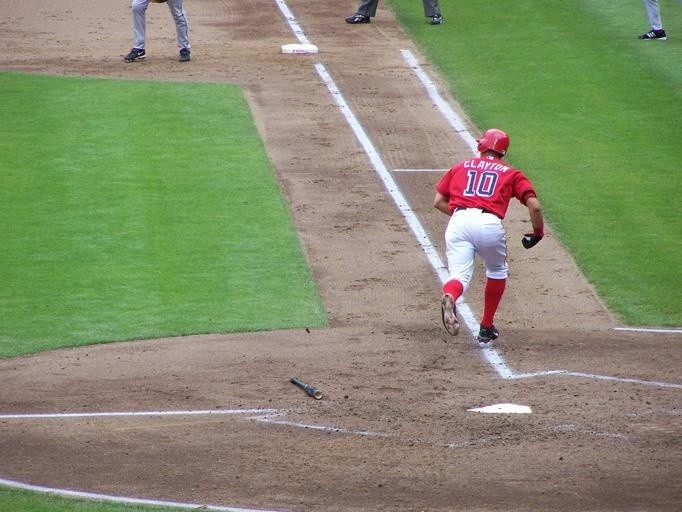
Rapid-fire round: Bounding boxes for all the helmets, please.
[476,128,510,157]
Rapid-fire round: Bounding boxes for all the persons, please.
[637,0,667,41]
[344,0,442,24]
[122,0,193,63]
[431,128,546,346]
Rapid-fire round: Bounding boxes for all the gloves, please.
[521,233,542,250]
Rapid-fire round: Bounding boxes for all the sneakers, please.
[345,13,370,24]
[431,15,444,25]
[478,326,499,343]
[440,294,461,336]
[178,48,192,62]
[124,47,147,62]
[638,29,668,41]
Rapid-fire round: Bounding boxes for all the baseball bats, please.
[290,378,322,400]
